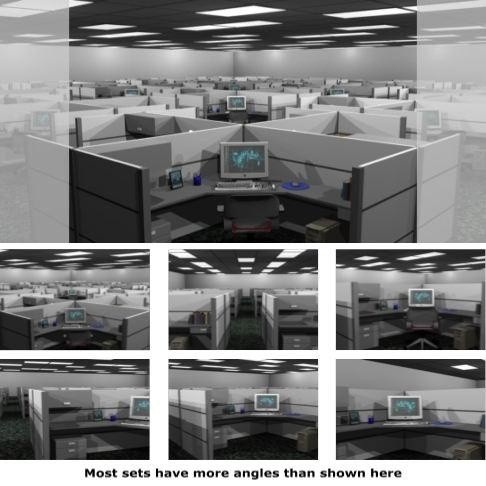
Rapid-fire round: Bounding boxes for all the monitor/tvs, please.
[129,395,150,420]
[407,288,435,308]
[254,394,280,412]
[64,307,87,325]
[330,88,344,95]
[68,289,78,295]
[124,88,140,96]
[219,141,269,181]
[226,96,247,111]
[387,396,423,419]
[30,109,62,131]
[414,108,442,129]
[229,83,240,90]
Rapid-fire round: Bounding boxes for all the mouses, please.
[291,181,300,187]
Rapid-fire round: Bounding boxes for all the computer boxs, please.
[454,327,479,349]
[296,427,318,452]
[305,217,349,243]
[453,443,483,460]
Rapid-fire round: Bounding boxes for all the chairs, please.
[403,308,441,349]
[61,325,93,349]
[208,186,283,239]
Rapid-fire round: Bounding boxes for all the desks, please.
[208,109,267,122]
[49,421,150,460]
[31,319,122,349]
[360,308,480,349]
[213,409,317,452]
[337,416,486,459]
[170,319,209,350]
[148,170,352,243]
[278,320,316,350]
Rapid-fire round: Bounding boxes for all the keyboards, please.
[121,420,149,427]
[384,420,422,426]
[61,324,85,328]
[250,412,277,416]
[214,181,271,193]
